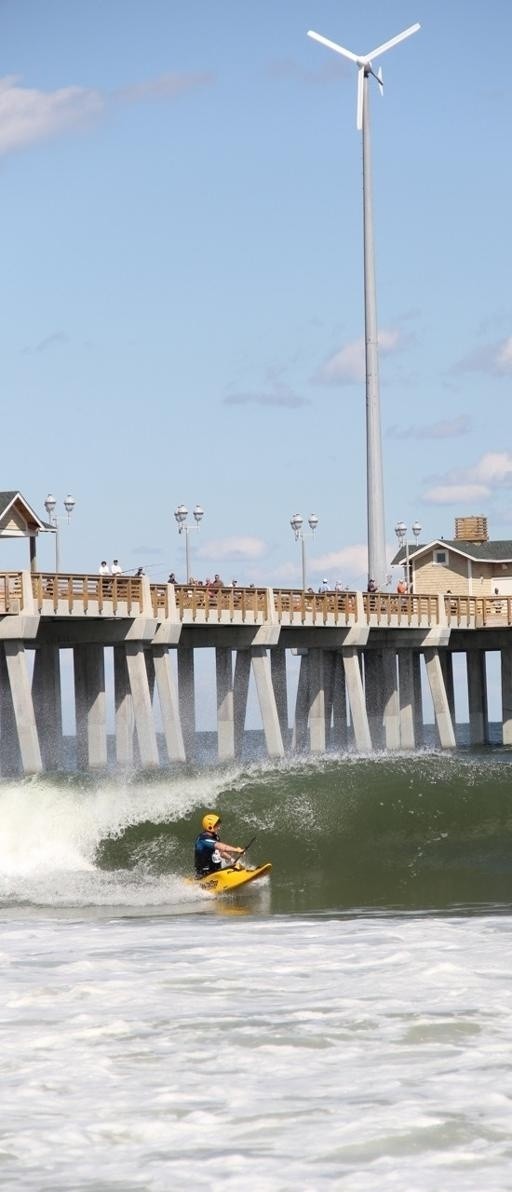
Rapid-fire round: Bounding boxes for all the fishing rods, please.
[118,562,159,575]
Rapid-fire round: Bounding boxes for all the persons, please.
[492,588,503,614]
[445,589,457,614]
[194,813,246,877]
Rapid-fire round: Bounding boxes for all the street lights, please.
[291,514,318,592]
[175,505,204,586]
[395,521,422,594]
[45,494,76,573]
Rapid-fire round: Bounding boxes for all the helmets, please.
[202,814,220,833]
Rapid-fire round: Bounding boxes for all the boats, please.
[188,863,273,894]
